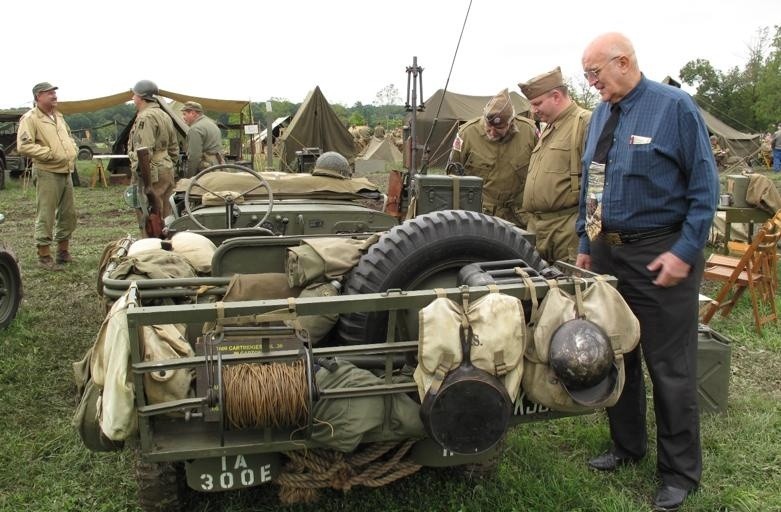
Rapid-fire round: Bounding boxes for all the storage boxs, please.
[414,173,484,214]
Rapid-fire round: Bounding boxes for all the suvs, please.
[0,109,38,188]
[76,164,620,510]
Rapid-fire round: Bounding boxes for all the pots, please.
[549,317,618,407]
[420,324,514,458]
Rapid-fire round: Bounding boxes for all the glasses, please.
[583,56,620,80]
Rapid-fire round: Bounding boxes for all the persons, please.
[574,31,720,509]
[763,131,772,170]
[710,135,725,167]
[179,101,229,179]
[16,81,80,271]
[518,68,594,273]
[127,79,181,240]
[445,89,542,232]
[771,123,781,172]
[350,119,402,146]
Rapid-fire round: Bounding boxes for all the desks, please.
[92,153,129,188]
[710,205,775,254]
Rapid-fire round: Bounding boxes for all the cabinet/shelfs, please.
[726,175,750,208]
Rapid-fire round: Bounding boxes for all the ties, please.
[584,102,619,242]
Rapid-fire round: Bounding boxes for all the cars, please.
[0,214,20,331]
[71,134,102,161]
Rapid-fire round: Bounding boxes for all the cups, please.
[720,195,731,206]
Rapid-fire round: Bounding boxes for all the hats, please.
[483,88,513,126]
[32,82,58,95]
[517,65,567,100]
[181,101,203,112]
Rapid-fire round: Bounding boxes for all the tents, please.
[357,135,402,161]
[403,90,535,169]
[244,115,294,154]
[279,86,361,173]
[658,77,761,169]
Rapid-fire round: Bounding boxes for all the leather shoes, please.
[39,254,64,272]
[652,483,687,511]
[56,250,73,264]
[587,449,623,470]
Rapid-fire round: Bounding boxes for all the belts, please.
[604,226,681,249]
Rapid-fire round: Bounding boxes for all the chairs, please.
[701,210,781,338]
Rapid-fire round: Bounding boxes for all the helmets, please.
[132,80,159,102]
[312,151,352,180]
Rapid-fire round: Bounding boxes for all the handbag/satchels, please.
[524,280,641,414]
[78,379,134,453]
[414,293,525,408]
[149,212,163,239]
